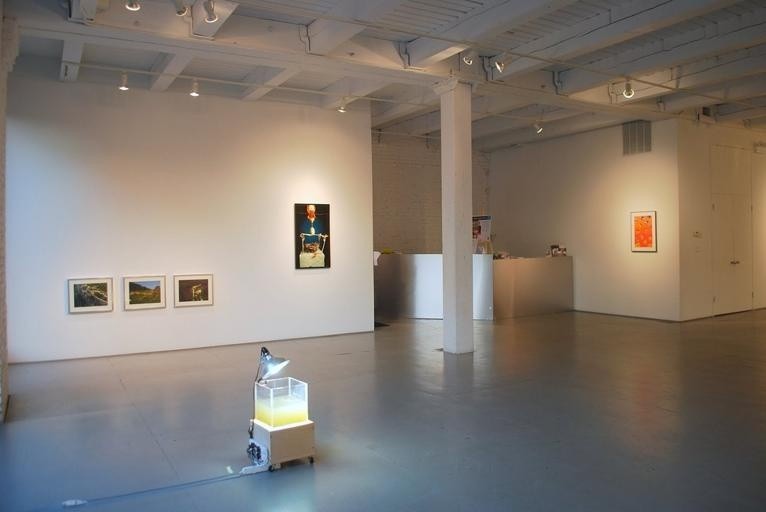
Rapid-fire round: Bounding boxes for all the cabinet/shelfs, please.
[254,376,316,470]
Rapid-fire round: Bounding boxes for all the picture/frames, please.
[630,210,657,253]
[67,274,214,314]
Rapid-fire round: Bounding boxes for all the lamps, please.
[250,346,289,456]
[338,50,634,134]
[118,0,217,97]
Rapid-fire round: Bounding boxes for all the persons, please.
[299,205,323,253]
